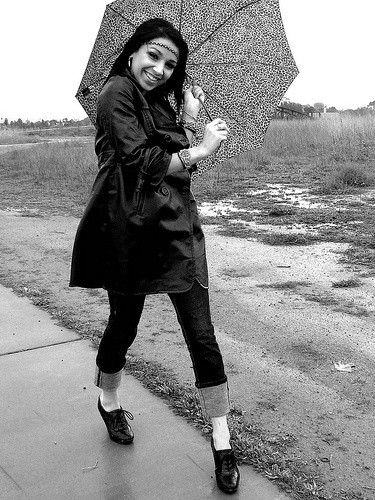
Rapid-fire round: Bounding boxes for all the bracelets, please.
[178,117,197,134]
[182,109,198,123]
[177,151,187,170]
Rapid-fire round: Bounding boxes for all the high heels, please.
[96,394,135,445]
[210,433,240,494]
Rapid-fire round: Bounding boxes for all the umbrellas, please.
[74,0,302,183]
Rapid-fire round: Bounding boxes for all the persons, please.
[67,16,241,496]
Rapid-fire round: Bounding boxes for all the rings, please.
[216,123,222,130]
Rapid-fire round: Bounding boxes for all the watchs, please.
[178,148,191,169]
